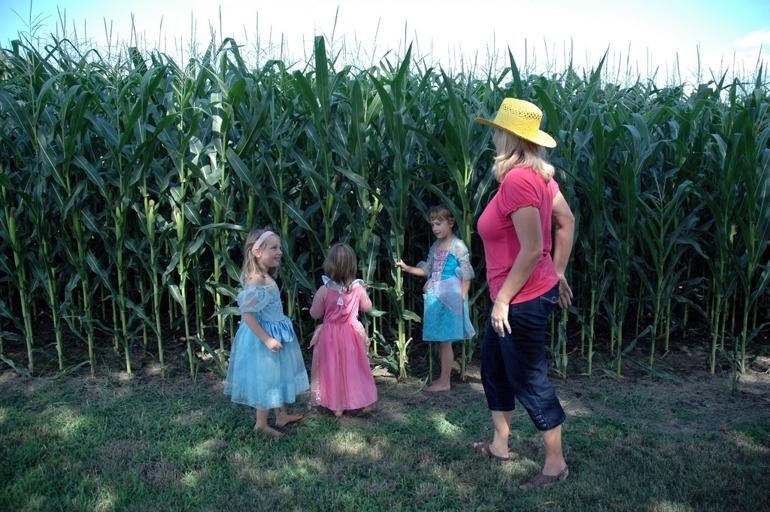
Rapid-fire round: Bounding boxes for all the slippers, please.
[465,439,510,462]
[517,466,573,493]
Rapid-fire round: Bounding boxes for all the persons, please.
[394,203,476,393]
[307,242,378,418]
[472,97,576,490]
[223,229,309,440]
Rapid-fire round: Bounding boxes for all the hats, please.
[475,97,557,148]
